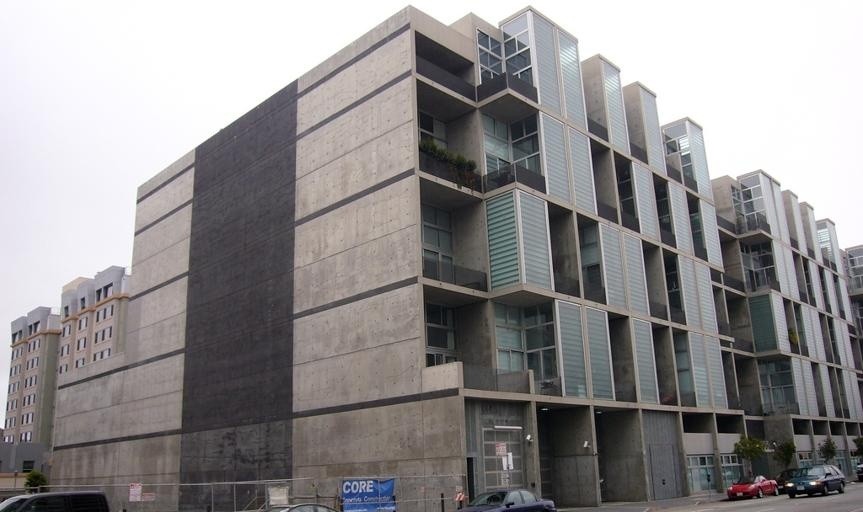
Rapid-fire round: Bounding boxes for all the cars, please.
[770,467,815,496]
[258,503,342,512]
[855,456,862,483]
[456,488,559,512]
[782,464,847,499]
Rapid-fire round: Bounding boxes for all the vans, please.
[0,489,114,512]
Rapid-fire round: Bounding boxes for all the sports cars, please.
[725,475,778,500]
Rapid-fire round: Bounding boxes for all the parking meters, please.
[706,471,713,500]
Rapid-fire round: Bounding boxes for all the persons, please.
[455,490,470,510]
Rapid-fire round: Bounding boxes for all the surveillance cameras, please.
[584,441,591,448]
[526,435,534,442]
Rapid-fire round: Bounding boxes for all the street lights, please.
[12,469,19,490]
